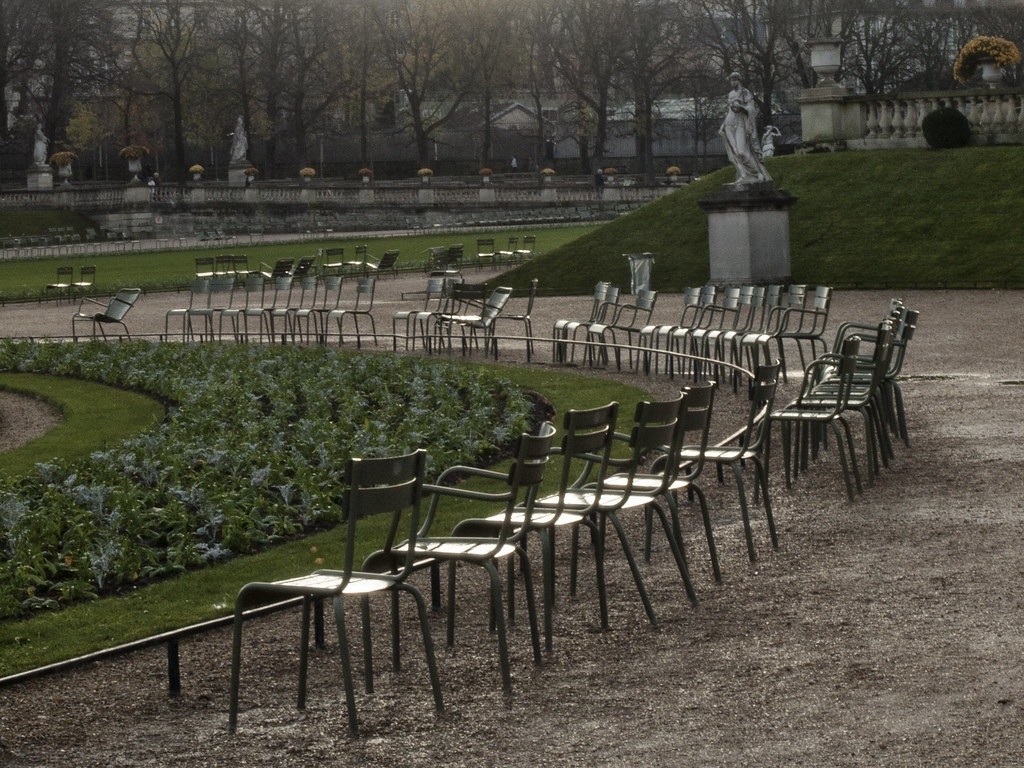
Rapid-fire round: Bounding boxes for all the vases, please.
[129,159,144,185]
[543,176,551,184]
[193,173,201,182]
[670,175,678,182]
[362,176,369,187]
[976,55,1006,88]
[483,176,489,183]
[304,176,312,187]
[607,175,614,181]
[422,175,429,183]
[246,175,255,188]
[57,163,73,185]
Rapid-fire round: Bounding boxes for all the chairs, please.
[46,266,74,306]
[71,288,141,342]
[72,265,98,305]
[162,236,921,738]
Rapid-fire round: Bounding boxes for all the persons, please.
[148,173,161,201]
[230,115,248,160]
[595,168,604,197]
[32,123,49,164]
[527,154,535,177]
[761,126,782,158]
[245,171,251,188]
[718,72,774,185]
[511,155,518,179]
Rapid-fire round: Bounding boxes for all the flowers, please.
[479,168,493,176]
[359,168,373,176]
[189,165,204,174]
[540,168,555,176]
[604,168,617,175]
[417,168,433,176]
[300,167,316,177]
[667,166,681,175]
[49,151,77,168]
[243,168,258,175]
[120,145,150,161]
[953,36,1021,85]
